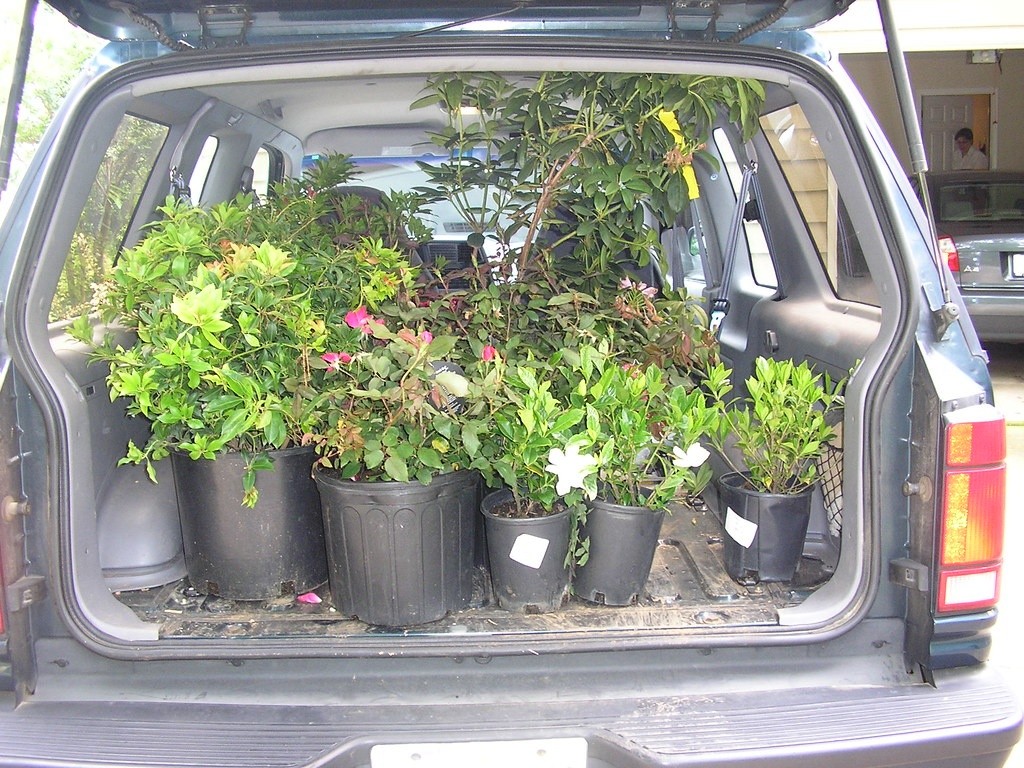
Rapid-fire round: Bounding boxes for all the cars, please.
[905,168,1024,347]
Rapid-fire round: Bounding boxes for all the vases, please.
[483,488,572,613]
[573,485,663,606]
[312,458,479,628]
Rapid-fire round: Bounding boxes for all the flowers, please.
[584,365,711,511]
[307,301,478,479]
[460,367,607,514]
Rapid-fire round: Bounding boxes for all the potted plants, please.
[706,356,861,587]
[64,193,325,605]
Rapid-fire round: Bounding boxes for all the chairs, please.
[318,186,439,297]
[941,201,975,218]
[525,192,665,300]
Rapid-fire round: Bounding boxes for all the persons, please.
[952,129,988,170]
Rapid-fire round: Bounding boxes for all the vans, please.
[2,0,1023,768]
